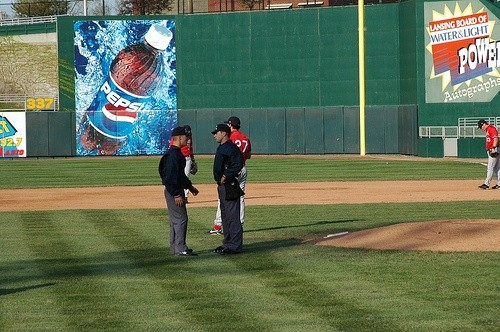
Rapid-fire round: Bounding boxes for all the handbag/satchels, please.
[223,177,245,201]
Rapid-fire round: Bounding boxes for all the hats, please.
[477,119,486,129]
[184,125,192,136]
[224,117,241,129]
[171,127,191,136]
[212,124,231,135]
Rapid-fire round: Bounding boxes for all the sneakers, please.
[188,249,193,252]
[492,185,500,189]
[208,226,222,233]
[478,184,489,189]
[176,250,198,256]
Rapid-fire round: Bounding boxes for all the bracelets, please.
[175,194,181,198]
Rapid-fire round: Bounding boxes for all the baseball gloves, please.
[489,151,500,158]
[190,162,198,175]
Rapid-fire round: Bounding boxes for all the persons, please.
[477,120,500,189]
[158,124,199,256]
[204,116,252,254]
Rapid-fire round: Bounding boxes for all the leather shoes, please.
[218,248,242,253]
[213,245,225,253]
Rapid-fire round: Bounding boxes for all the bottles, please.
[79,25,173,155]
